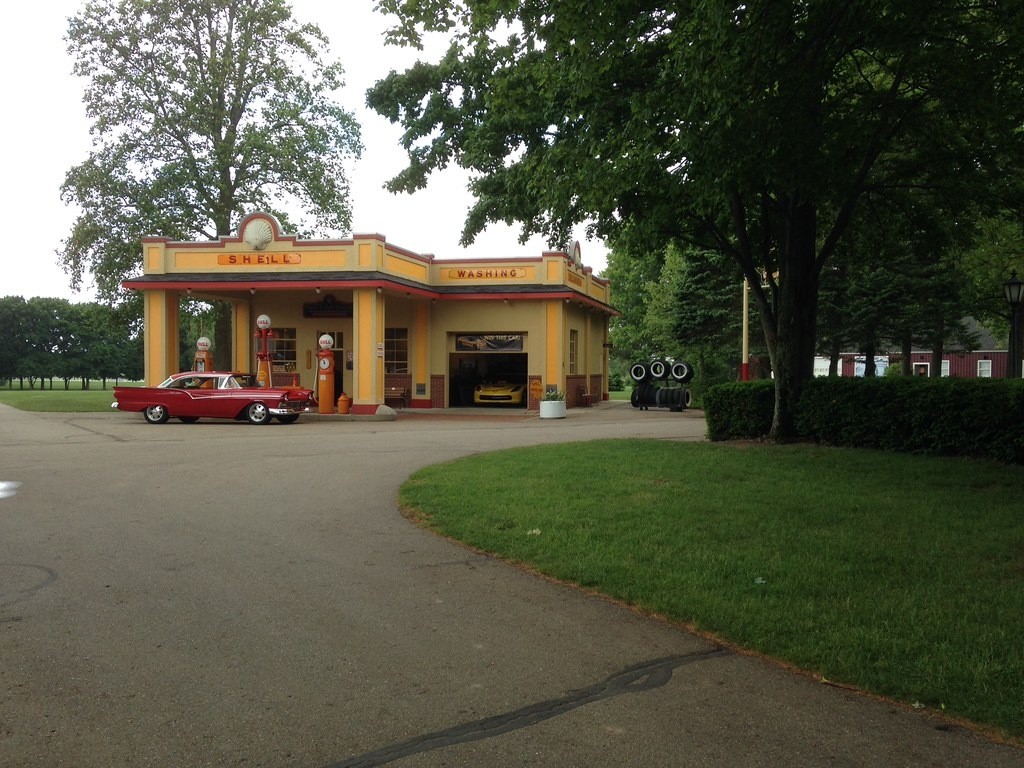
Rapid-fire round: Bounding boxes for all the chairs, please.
[579,385,600,407]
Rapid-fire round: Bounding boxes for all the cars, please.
[474,373,527,407]
[111,371,314,425]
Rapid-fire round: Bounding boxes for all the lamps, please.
[250,288,255,294]
[186,288,192,294]
[503,298,508,304]
[565,298,570,303]
[377,287,382,294]
[315,287,321,294]
[579,302,582,307]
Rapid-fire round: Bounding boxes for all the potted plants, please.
[539,390,567,418]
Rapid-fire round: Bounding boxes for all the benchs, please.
[385,387,407,409]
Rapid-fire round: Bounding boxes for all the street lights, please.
[1002,268,1024,378]
